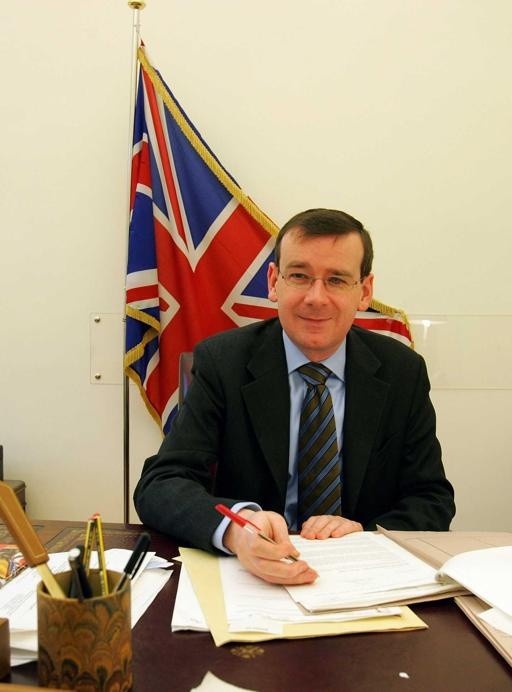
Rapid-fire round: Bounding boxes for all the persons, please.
[133,208,457,585]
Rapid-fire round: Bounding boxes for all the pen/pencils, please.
[113,532,151,594]
[68,512,109,599]
[215,502,319,578]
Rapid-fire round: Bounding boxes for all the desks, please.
[1,519,512,692]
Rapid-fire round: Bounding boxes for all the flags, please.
[123,39,414,435]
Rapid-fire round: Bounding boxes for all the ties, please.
[297,362,341,534]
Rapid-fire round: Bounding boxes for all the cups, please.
[37,571,132,692]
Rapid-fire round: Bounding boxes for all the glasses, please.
[278,270,368,295]
